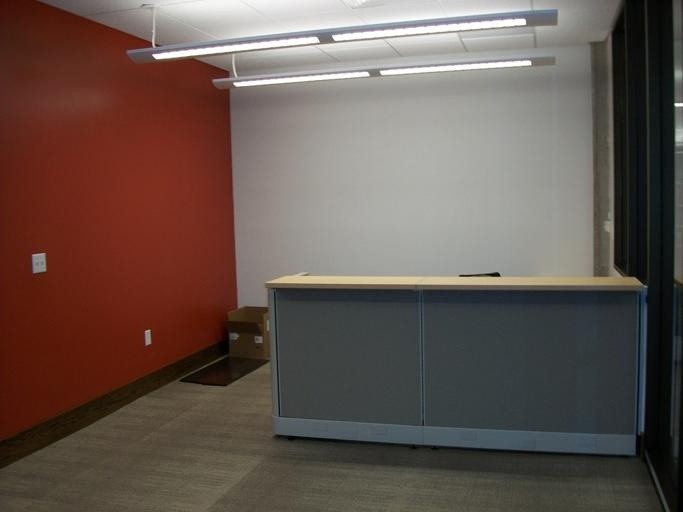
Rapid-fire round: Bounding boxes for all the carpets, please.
[178,352,268,388]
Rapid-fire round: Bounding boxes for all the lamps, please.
[122,0,557,73]
[210,26,555,92]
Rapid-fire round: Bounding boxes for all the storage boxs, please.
[225,301,272,361]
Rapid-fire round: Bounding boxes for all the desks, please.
[262,269,643,460]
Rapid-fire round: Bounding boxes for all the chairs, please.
[456,272,502,278]
